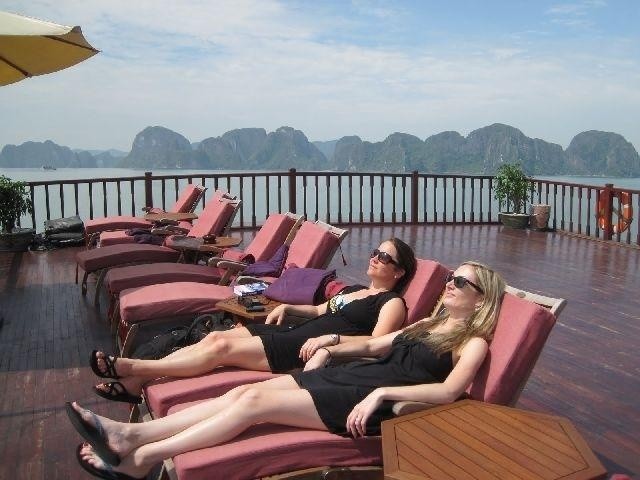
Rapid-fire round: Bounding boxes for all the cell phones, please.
[246,305,264,312]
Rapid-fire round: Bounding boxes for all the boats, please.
[41,164,56,171]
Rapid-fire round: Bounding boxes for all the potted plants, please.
[492,161,539,229]
[0,175,36,252]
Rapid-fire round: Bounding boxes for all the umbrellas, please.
[1,10,101,86]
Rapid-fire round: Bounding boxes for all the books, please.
[234,282,268,296]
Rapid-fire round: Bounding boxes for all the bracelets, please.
[331,333,340,345]
[322,347,331,358]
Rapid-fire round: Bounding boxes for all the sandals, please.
[65,401,147,480]
[88,349,143,404]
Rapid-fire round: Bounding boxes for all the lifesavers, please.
[595,191,633,234]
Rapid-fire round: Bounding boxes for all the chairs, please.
[157,286,568,480]
[104,208,306,332]
[143,256,452,423]
[110,219,352,361]
[74,186,245,295]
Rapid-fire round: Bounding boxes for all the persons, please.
[88,238,418,405]
[65,260,503,480]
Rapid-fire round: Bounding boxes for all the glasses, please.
[445,271,482,292]
[370,249,400,267]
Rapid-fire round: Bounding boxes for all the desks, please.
[183,262,352,356]
[382,397,610,479]
